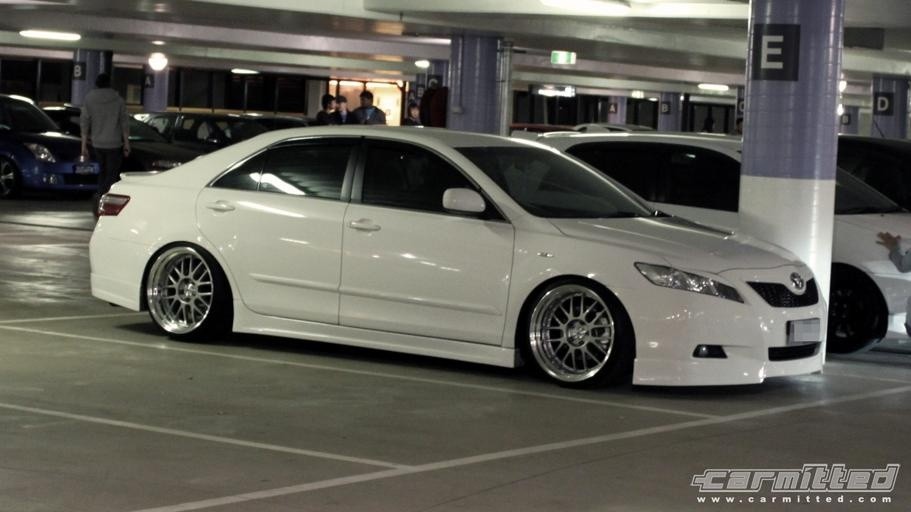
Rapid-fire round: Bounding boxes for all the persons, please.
[79,74,132,190]
[328,96,354,126]
[317,94,338,126]
[402,102,420,126]
[348,91,388,125]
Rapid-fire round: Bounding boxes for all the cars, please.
[0,94,911,388]
